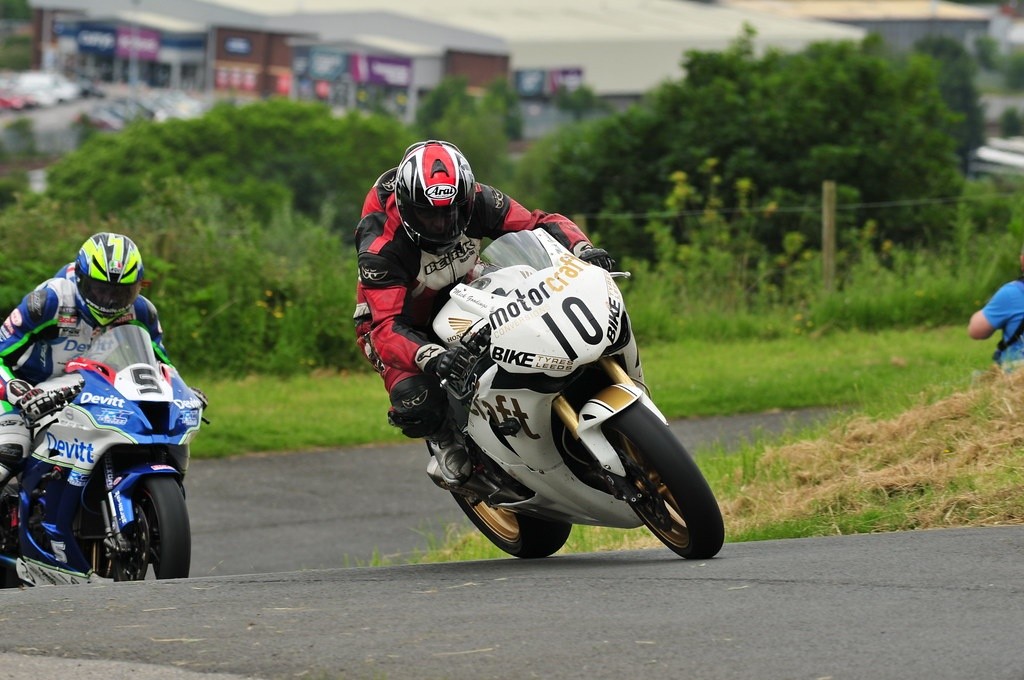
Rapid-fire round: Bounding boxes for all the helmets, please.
[73,232,145,326]
[394,139,476,257]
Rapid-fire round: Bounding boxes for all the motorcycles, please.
[425,228,725,559]
[0,325,209,588]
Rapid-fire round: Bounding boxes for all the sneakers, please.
[426,425,473,487]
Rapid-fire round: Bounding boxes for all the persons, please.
[967,243,1024,363]
[353,141,607,485]
[0,233,208,555]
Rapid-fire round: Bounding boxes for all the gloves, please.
[571,240,616,273]
[4,378,55,418]
[415,344,474,381]
[187,385,208,414]
